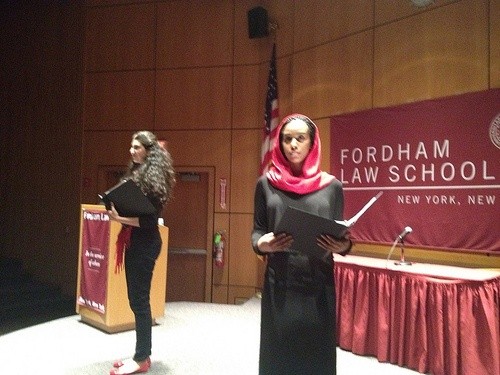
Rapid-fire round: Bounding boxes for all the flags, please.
[260,44,281,177]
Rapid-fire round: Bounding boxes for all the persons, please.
[106,130,176,375]
[251,114,353,375]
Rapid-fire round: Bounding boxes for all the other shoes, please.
[114,357,150,367]
[110,359,149,375]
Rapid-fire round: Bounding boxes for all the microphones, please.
[395,227,412,243]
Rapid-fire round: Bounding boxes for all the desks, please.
[332,252,500,375]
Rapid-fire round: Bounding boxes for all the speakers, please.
[248,7,267,37]
